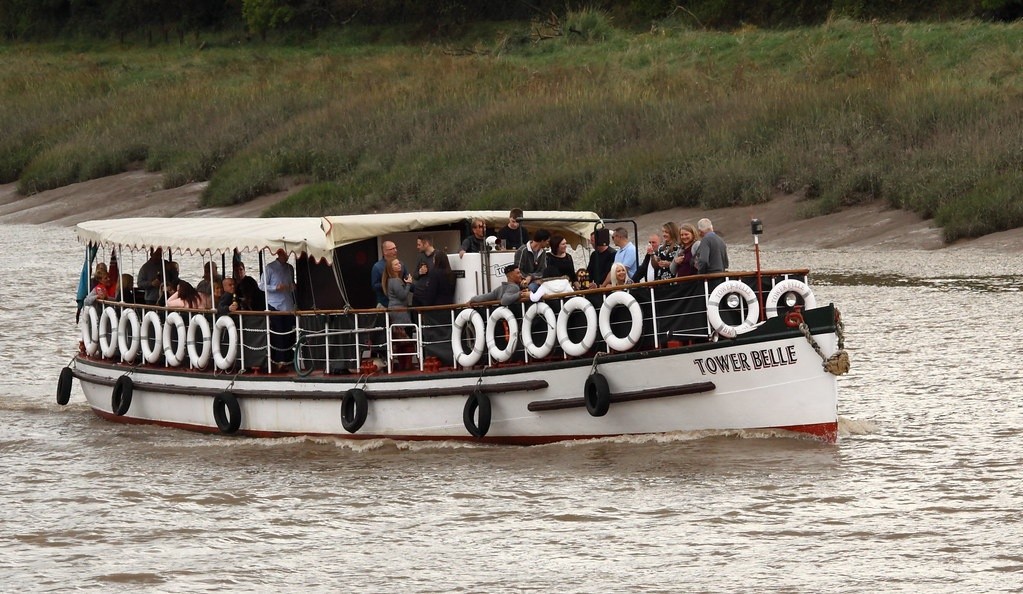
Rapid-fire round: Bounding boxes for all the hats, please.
[505,265,518,274]
[549,236,565,252]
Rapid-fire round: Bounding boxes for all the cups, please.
[501,239,506,250]
[280,281,285,290]
[289,281,295,291]
[404,274,409,280]
[421,263,426,271]
[676,248,685,257]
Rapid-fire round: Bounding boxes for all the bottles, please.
[155,271,160,281]
[579,271,590,290]
[647,243,653,254]
[232,293,238,311]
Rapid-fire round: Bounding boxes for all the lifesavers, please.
[598,290,643,352]
[117,308,140,361]
[162,312,186,368]
[186,313,211,370]
[556,296,598,356]
[765,278,817,320]
[211,315,238,370]
[340,388,368,433]
[213,392,241,434]
[81,305,99,355]
[56,367,73,405]
[140,310,163,363]
[485,306,519,363]
[583,373,610,417]
[452,308,485,367]
[521,302,557,359]
[111,375,133,416]
[463,394,491,438]
[707,279,760,338]
[99,306,119,358]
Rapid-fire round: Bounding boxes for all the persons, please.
[459,216,491,258]
[576,227,637,285]
[466,229,576,306]
[371,234,455,367]
[633,218,729,283]
[81,247,297,370]
[496,208,529,250]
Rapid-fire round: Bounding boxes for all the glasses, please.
[612,235,617,240]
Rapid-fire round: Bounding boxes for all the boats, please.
[71,208,854,447]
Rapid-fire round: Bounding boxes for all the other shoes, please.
[278,364,288,373]
